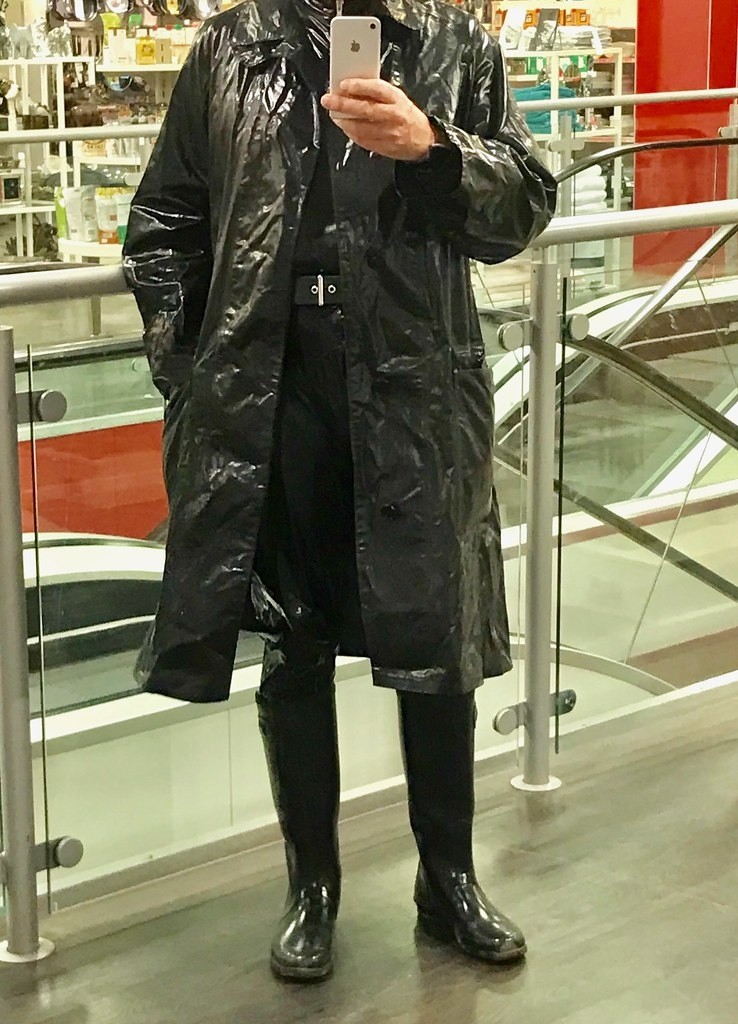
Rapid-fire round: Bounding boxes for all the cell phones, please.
[329,16,381,119]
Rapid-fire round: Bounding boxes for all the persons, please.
[118,0,557,979]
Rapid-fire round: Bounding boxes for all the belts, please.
[289,274,343,308]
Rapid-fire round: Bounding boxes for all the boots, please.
[254,680,342,979]
[398,687,527,961]
